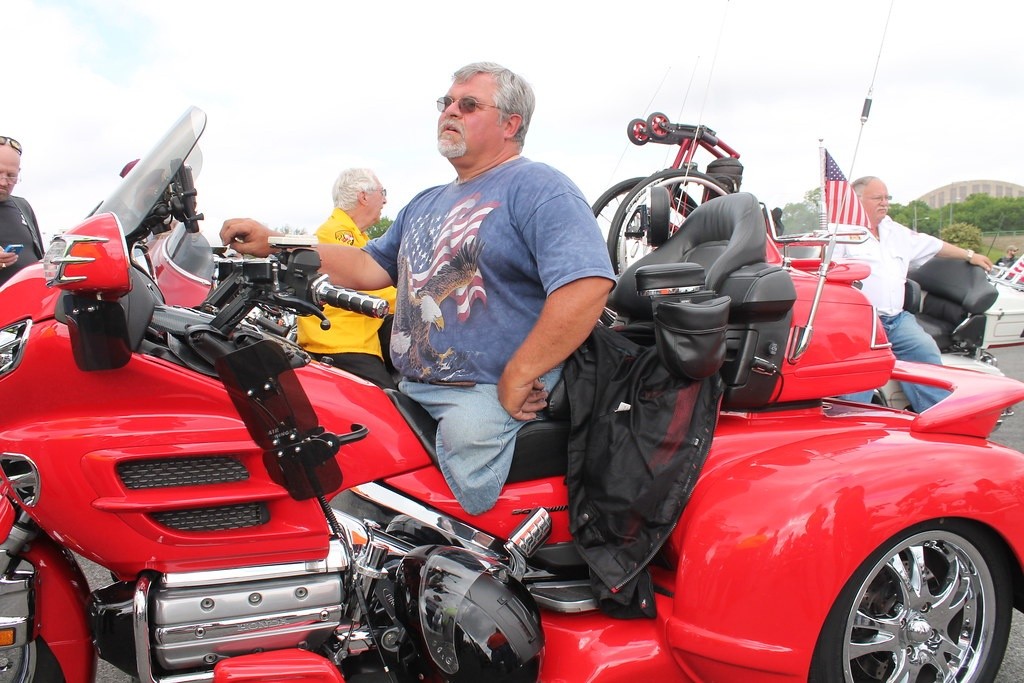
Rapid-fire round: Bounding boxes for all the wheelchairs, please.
[589,111,745,278]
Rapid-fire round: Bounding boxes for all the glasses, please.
[436,96,512,113]
[857,195,892,202]
[0,172,21,185]
[368,189,387,197]
[0,136,22,156]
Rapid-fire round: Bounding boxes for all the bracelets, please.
[966,249,974,262]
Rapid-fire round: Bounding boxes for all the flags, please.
[824,147,872,229]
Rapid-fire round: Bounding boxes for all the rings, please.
[2,263,6,268]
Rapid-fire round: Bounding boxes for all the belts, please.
[402,376,476,387]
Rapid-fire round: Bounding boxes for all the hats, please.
[1005,246,1019,253]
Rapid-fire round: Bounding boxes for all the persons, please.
[294,162,397,389]
[0,135,46,287]
[994,245,1020,272]
[219,61,619,517]
[811,176,993,414]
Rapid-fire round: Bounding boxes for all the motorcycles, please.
[0,106,1024,682]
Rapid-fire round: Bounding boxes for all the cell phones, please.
[3,243,25,254]
